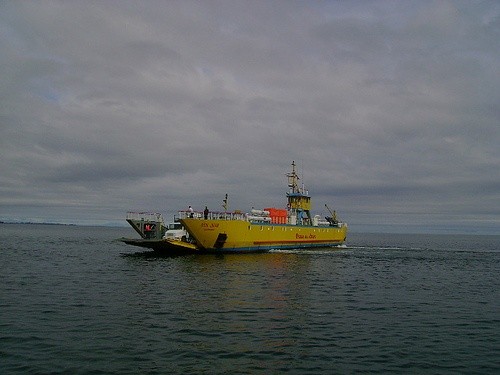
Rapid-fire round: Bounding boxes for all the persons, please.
[145,222,151,240]
[204,206,209,219]
[189,206,194,219]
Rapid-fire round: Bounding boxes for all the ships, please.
[120,160,348,253]
